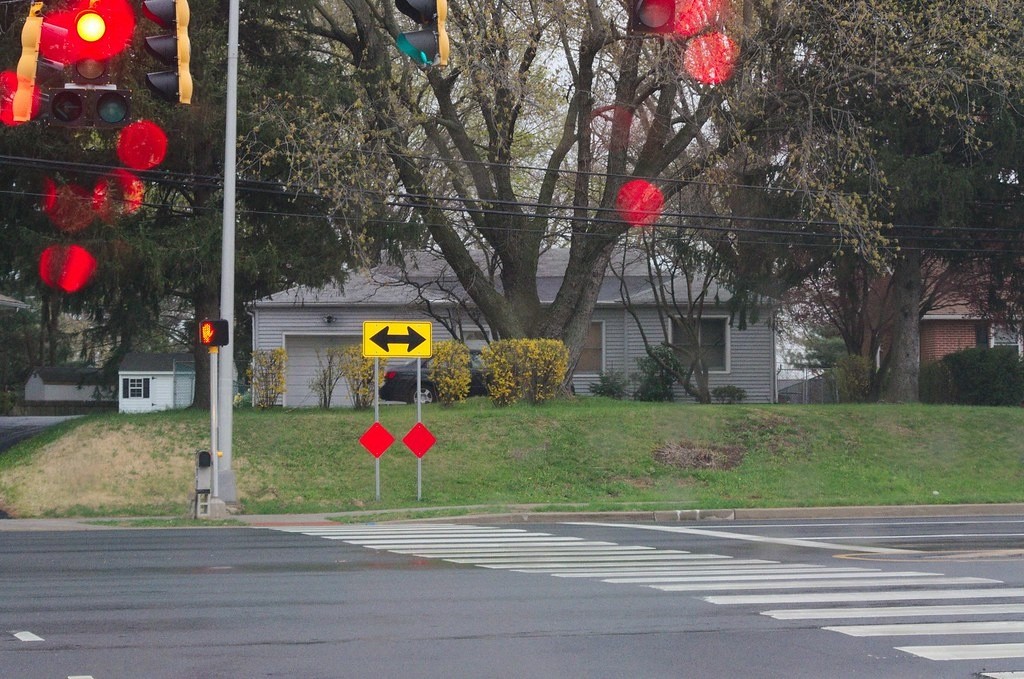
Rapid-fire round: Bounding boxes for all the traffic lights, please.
[198,319,229,349]
[395,0,450,68]
[67,0,115,84]
[11,2,68,124]
[627,0,675,34]
[46,86,133,128]
[139,0,194,106]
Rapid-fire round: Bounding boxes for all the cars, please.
[378,351,501,406]
